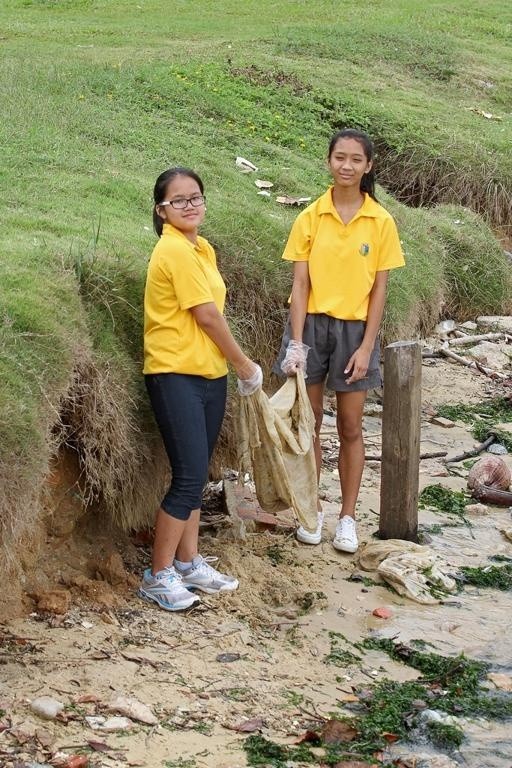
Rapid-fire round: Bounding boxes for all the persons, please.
[282,131,405,555]
[136,165,269,614]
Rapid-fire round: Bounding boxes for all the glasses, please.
[159,196,206,210]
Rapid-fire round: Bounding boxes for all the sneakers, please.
[136,567,199,612]
[330,514,359,554]
[295,505,325,545]
[173,556,241,595]
